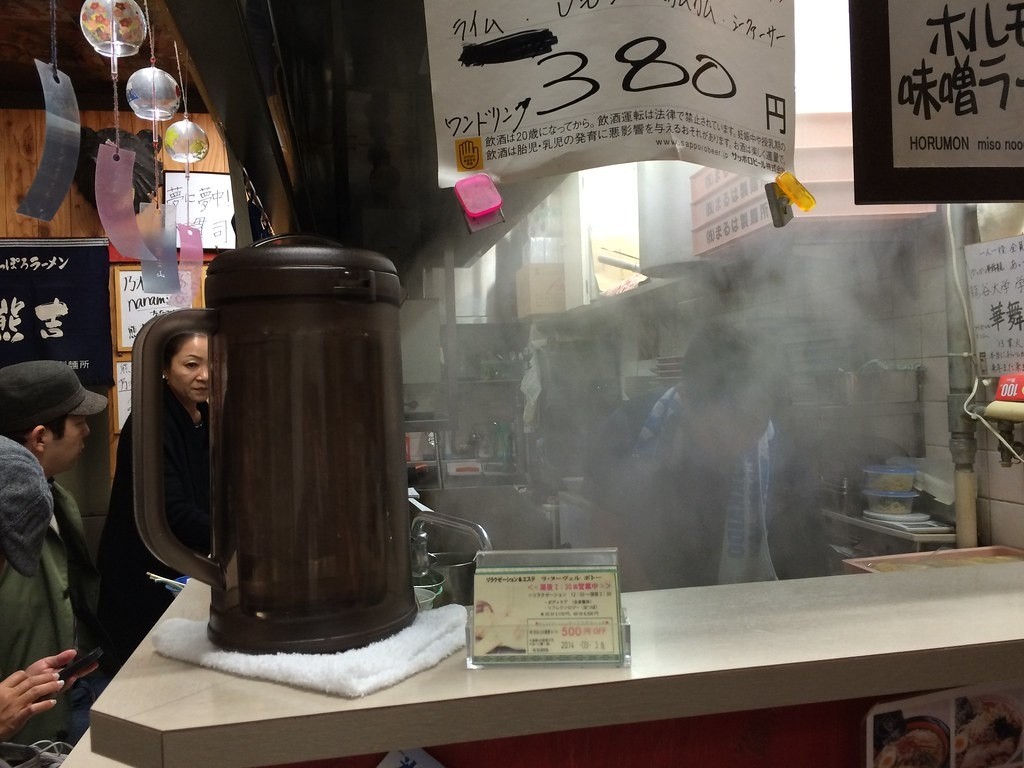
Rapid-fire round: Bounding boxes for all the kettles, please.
[132,232,418,655]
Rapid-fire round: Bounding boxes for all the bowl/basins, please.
[874,716,951,768]
[413,569,447,613]
[959,696,1024,768]
[860,465,920,516]
[165,576,192,598]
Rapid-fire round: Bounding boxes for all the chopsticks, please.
[146,573,186,588]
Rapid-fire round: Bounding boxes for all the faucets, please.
[411,512,493,576]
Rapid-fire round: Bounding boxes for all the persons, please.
[0,435,76,740]
[0,360,110,739]
[101,330,210,629]
[594,324,834,589]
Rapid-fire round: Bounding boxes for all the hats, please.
[0,360,108,433]
[0,436,53,577]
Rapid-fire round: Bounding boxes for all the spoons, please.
[494,350,525,360]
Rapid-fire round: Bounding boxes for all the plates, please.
[780,336,850,404]
[623,356,687,399]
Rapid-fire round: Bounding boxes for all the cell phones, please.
[57,646,104,681]
